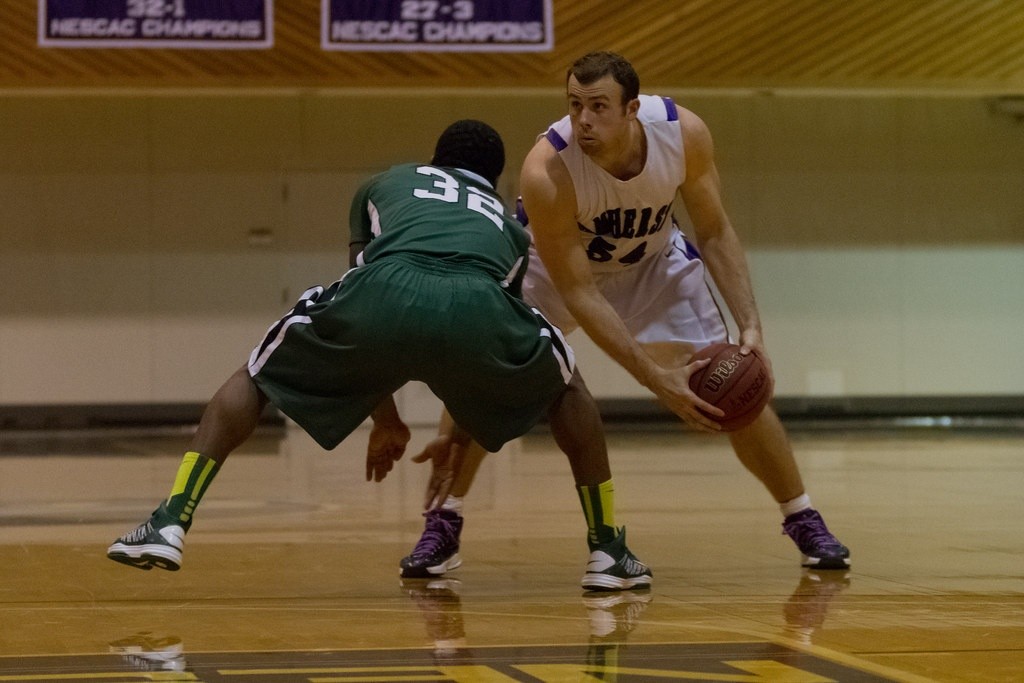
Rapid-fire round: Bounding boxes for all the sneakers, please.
[782,510,851,568]
[581,526,654,591]
[107,498,193,570]
[399,508,462,578]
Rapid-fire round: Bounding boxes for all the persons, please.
[105,117,656,595]
[398,51,854,579]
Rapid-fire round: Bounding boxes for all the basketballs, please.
[685,343,771,432]
